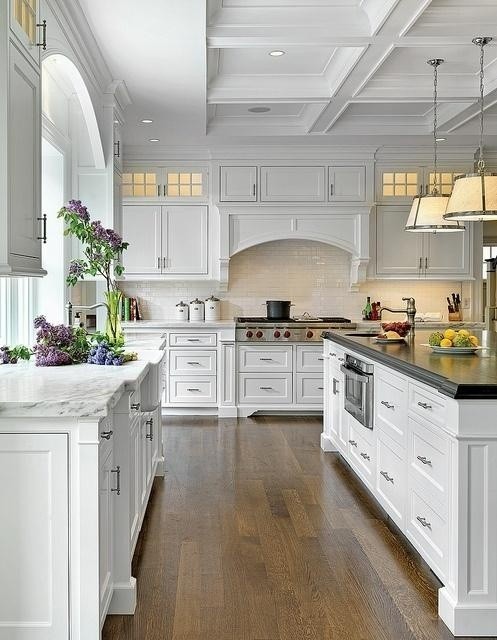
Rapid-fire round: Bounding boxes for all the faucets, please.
[377,297,416,336]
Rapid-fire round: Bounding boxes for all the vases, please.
[103,289,124,344]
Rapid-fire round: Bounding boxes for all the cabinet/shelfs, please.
[2,401,119,639]
[369,204,477,280]
[0,0,46,277]
[214,161,375,208]
[323,341,455,611]
[235,343,326,417]
[120,340,167,617]
[372,163,475,205]
[126,330,221,413]
[111,121,124,281]
[120,166,208,197]
[118,199,213,282]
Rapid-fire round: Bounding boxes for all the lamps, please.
[439,33,496,221]
[403,58,466,236]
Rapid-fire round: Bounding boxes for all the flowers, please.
[0,314,136,369]
[56,199,129,291]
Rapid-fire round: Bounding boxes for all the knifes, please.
[445,292,460,313]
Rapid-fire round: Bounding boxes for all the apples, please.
[453,336,471,347]
[429,332,444,346]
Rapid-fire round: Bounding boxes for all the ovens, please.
[339,352,374,430]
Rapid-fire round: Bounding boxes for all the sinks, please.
[345,333,380,337]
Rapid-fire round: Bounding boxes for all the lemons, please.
[459,329,469,337]
[440,339,452,347]
[468,336,479,347]
[444,329,458,341]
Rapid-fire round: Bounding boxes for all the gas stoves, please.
[234,314,356,342]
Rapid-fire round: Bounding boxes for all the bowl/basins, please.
[404,312,444,321]
[380,322,413,336]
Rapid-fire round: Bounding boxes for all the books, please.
[119,295,144,321]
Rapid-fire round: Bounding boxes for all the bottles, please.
[174,295,220,322]
[361,295,381,320]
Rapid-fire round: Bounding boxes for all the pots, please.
[261,300,295,320]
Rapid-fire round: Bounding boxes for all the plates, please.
[370,336,405,344]
[420,343,491,354]
